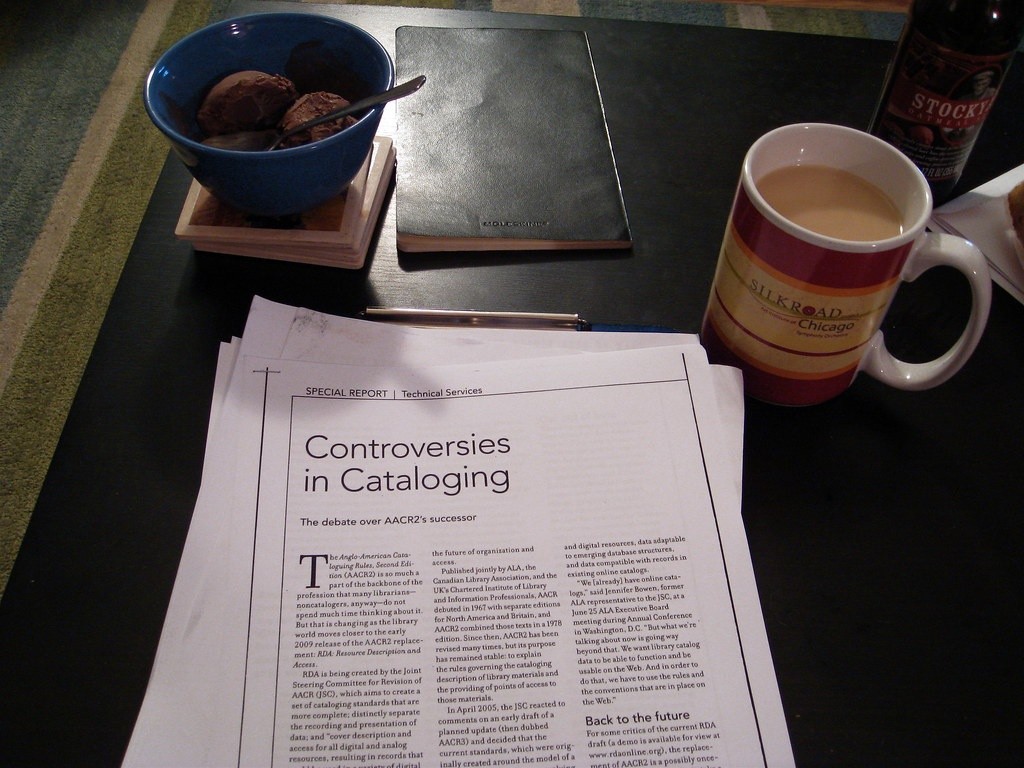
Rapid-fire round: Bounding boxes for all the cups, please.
[700,124,993,408]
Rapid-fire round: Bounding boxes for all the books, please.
[395,25,633,252]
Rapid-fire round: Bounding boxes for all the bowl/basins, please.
[144,12,394,213]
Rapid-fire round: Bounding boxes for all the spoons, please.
[251,76,427,151]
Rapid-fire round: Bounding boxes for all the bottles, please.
[870,0,1024,199]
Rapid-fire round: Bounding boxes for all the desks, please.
[2,1,1024,768]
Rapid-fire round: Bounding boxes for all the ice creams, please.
[197,69,361,153]
